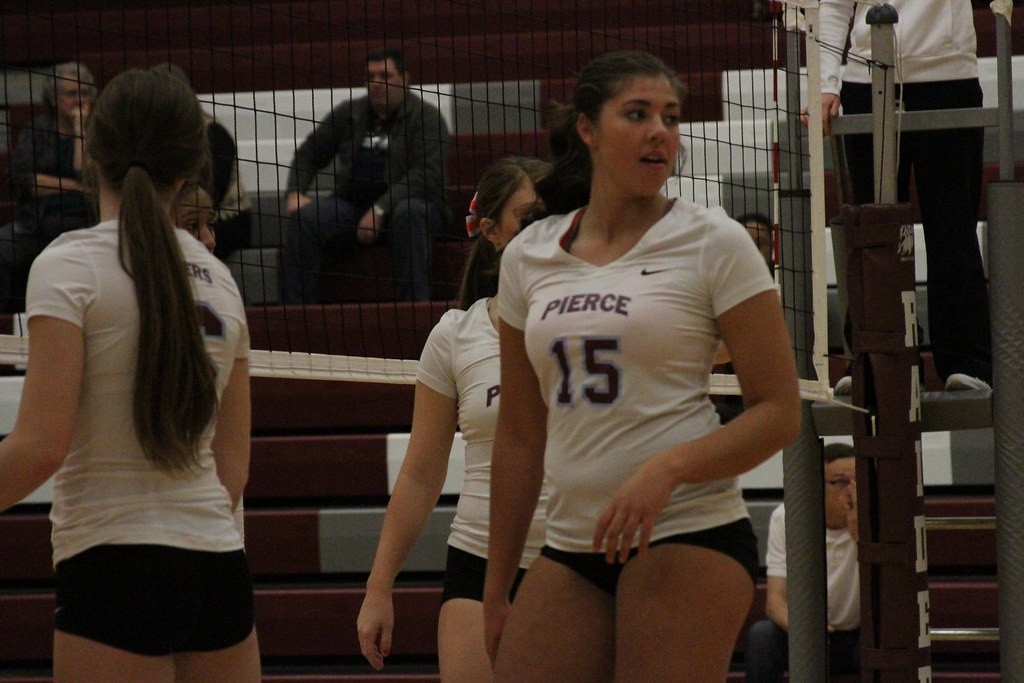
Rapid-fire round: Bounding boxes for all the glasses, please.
[824,477,851,490]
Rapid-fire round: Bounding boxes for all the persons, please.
[0,49,866,683]
[801,0,995,395]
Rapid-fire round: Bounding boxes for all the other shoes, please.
[834,374,852,397]
[944,371,992,392]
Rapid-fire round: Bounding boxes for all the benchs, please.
[216,195,288,304]
[453,82,544,183]
[723,69,844,140]
[195,85,453,193]
[662,120,776,218]
[544,71,722,132]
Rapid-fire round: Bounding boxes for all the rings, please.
[805,111,809,115]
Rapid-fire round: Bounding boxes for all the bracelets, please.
[372,205,385,216]
